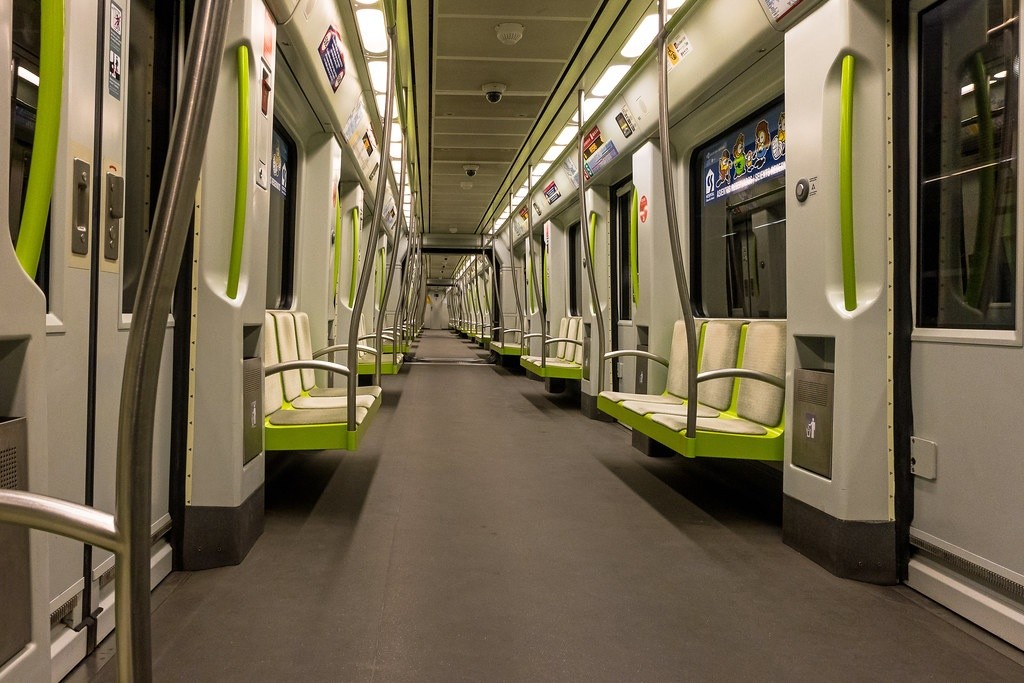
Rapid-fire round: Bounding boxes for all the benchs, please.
[448,318,494,349]
[489,319,530,356]
[264,311,384,452]
[520,317,585,380]
[380,318,424,353]
[356,314,404,375]
[596,317,787,460]
